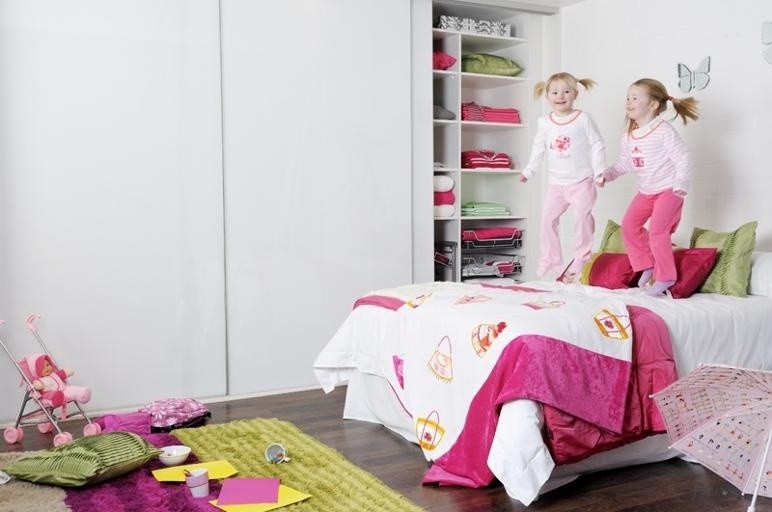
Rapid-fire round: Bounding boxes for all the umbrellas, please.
[648,363,772,512]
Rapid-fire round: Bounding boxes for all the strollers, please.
[0,314,102,448]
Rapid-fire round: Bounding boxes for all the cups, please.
[264,443,291,464]
[184,469,209,498]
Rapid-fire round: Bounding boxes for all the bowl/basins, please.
[158,445,191,467]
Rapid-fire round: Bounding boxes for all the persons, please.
[520,72,607,279]
[595,78,700,297]
[15,353,91,408]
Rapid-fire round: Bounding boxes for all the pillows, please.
[579,217,772,299]
[4,431,164,487]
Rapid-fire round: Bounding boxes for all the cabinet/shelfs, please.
[432,0,560,283]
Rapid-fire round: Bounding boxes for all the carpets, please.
[1,417,424,512]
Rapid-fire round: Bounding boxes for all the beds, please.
[358,279,772,496]
